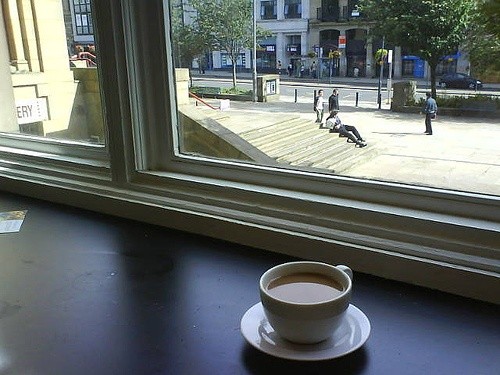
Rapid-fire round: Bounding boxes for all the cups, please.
[260,261,352,344]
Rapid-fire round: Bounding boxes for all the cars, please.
[439,71,483,90]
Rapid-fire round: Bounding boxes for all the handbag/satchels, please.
[430,113,436,119]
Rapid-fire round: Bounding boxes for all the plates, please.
[241,302,370,360]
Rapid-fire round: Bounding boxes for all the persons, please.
[314,90,324,123]
[326,110,367,147]
[422,92,437,135]
[288,61,317,78]
[329,88,339,116]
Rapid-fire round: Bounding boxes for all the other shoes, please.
[426,133,432,135]
[360,143,368,148]
[424,131,429,133]
[358,138,365,142]
